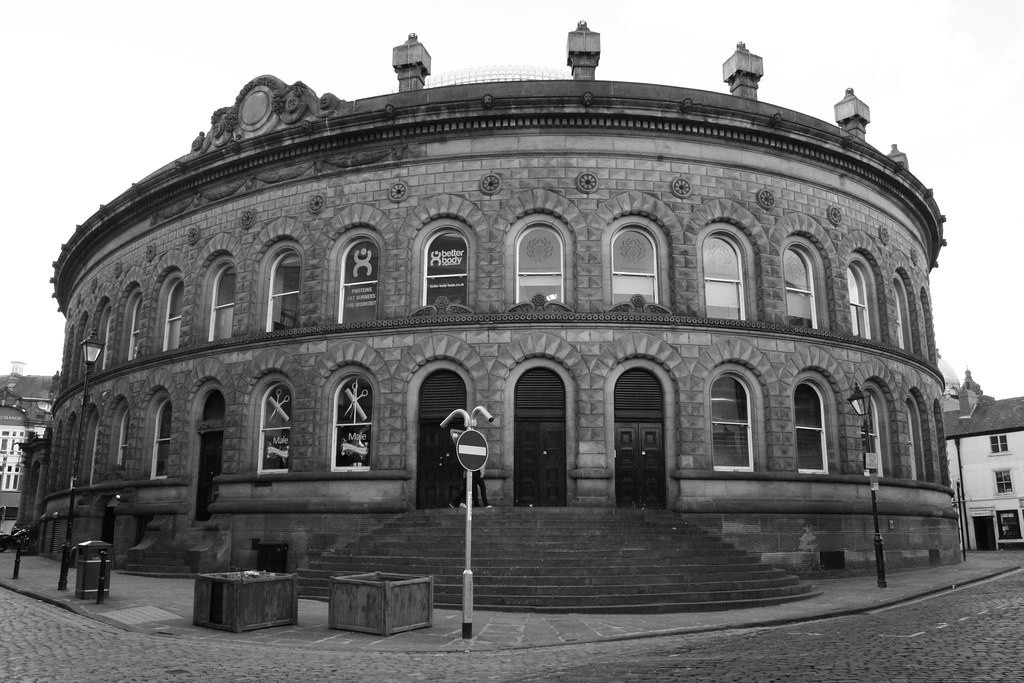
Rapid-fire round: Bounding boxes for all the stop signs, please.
[456,429,489,471]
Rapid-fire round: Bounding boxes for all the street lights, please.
[847,381,888,588]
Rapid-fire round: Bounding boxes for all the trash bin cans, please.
[258,542,289,573]
[75,541,113,599]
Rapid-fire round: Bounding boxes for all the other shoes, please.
[448,503,454,509]
[459,502,466,508]
[485,505,492,508]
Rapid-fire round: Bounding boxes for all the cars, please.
[0,530,11,552]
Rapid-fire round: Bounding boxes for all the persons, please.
[443,454,492,508]
